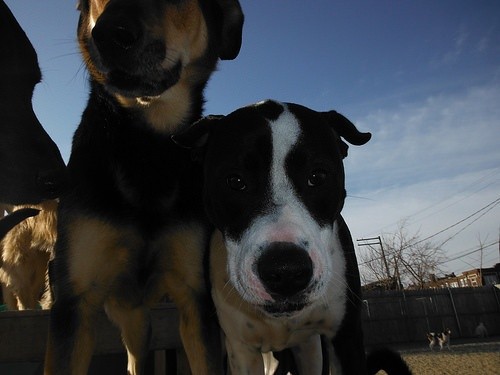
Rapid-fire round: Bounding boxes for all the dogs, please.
[474,322,488,339]
[0,0,413,375]
[427,328,451,352]
